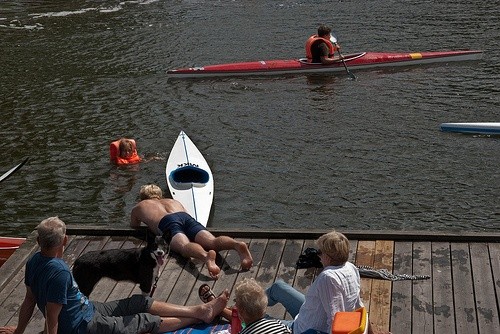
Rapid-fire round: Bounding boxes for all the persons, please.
[198,233,374,334]
[309,25,344,64]
[130,185,252,280]
[0,216,231,334]
[117,140,137,160]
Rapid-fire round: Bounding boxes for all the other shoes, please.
[296,247,323,268]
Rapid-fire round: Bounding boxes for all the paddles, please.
[330,35,356,79]
[0,156,30,183]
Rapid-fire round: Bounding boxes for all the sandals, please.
[198,283,216,304]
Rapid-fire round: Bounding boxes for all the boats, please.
[440,121,500,137]
[164,130,214,230]
[168,51,484,77]
[0,157,29,183]
